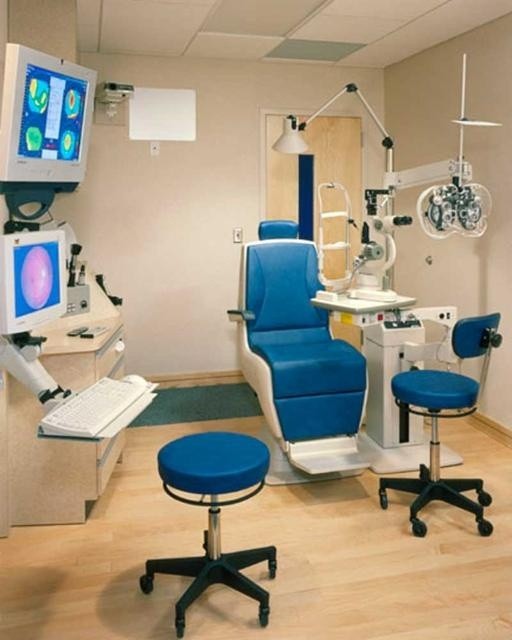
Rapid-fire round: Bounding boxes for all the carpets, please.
[130,379,264,425]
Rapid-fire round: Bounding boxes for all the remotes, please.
[68,327,87,336]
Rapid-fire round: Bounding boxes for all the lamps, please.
[273,81,395,173]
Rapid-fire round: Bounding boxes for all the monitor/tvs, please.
[0,229,67,335]
[0,43,98,184]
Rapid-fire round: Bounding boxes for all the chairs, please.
[379,313,501,510]
[234,217,367,475]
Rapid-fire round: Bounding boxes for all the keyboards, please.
[40,377,148,438]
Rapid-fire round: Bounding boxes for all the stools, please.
[142,432,275,637]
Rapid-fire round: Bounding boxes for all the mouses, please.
[122,374,148,387]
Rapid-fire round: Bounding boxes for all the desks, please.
[0,313,130,524]
